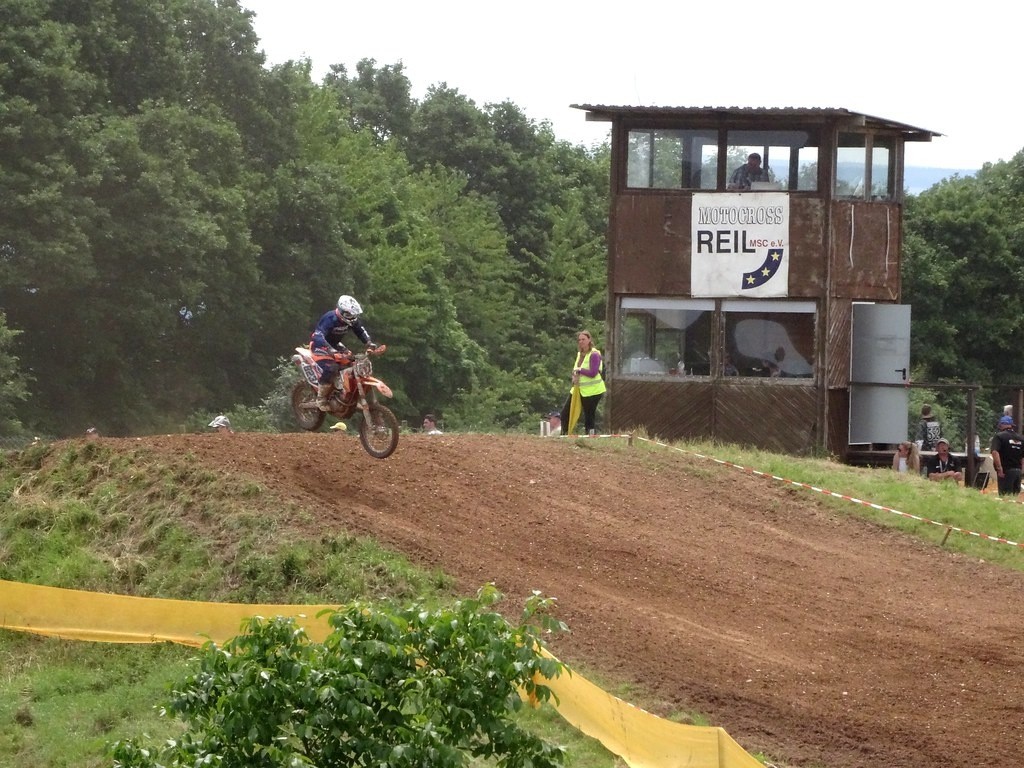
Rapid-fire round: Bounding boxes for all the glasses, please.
[898,448,906,452]
[341,310,359,320]
[547,417,556,421]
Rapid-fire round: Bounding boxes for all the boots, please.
[315,382,332,412]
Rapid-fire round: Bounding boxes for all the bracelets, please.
[996,465,1002,469]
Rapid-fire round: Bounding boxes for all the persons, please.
[727,153,768,191]
[309,295,377,411]
[915,403,943,472]
[892,441,921,476]
[990,416,1024,496]
[544,411,563,437]
[328,422,348,435]
[979,446,988,453]
[208,416,233,433]
[85,428,97,438]
[423,413,443,434]
[724,351,804,378]
[927,437,962,485]
[560,331,608,436]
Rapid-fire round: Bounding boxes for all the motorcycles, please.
[291,342,400,459]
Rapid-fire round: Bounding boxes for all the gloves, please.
[366,341,378,351]
[329,346,339,358]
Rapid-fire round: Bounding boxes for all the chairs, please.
[973,472,990,491]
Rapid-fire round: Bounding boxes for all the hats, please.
[545,410,561,417]
[936,437,949,445]
[329,422,347,431]
[999,416,1013,425]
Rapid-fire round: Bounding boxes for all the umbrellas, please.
[567,369,582,435]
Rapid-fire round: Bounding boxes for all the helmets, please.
[207,416,229,427]
[336,295,364,327]
